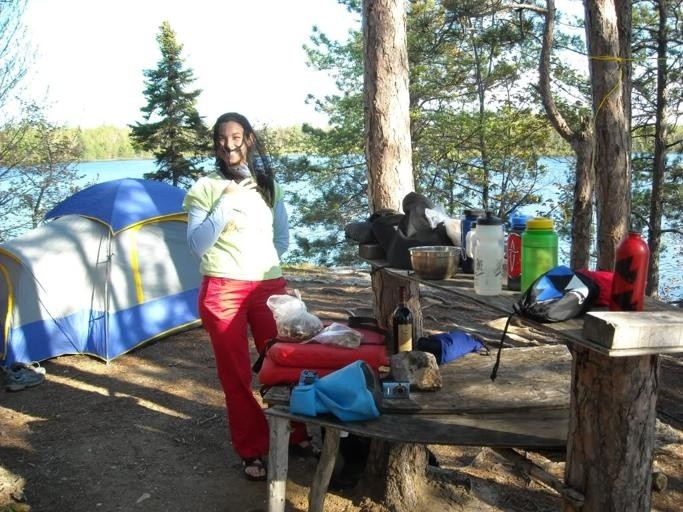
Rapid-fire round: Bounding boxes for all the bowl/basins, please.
[407,244,461,280]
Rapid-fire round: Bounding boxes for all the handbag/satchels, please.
[491,265,597,382]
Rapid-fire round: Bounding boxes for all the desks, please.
[261,344,573,512]
[354,252,683,357]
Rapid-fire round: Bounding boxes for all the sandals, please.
[287,440,321,459]
[242,457,267,481]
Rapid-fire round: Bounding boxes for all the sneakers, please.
[6,361,46,392]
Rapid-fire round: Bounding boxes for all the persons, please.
[181,111,321,481]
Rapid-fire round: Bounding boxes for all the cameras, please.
[381,381,410,399]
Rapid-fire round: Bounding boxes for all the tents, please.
[0,176,203,369]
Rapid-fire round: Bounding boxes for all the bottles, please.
[392,286,414,353]
[521,217,558,292]
[459,208,485,274]
[472,209,504,296]
[507,216,531,291]
[608,233,650,315]
